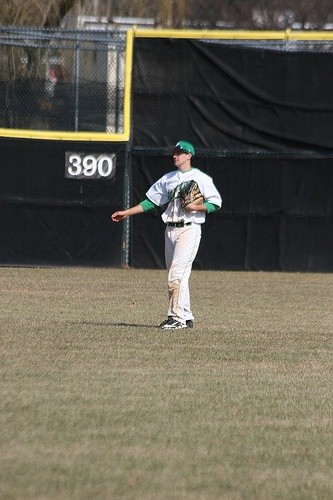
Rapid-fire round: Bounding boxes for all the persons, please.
[111,141,222,329]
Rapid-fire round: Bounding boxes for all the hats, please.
[170,139,195,158]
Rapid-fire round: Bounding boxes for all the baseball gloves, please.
[179,180,205,215]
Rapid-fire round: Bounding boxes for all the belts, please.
[169,222,191,228]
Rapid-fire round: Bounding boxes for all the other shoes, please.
[159,318,186,330]
